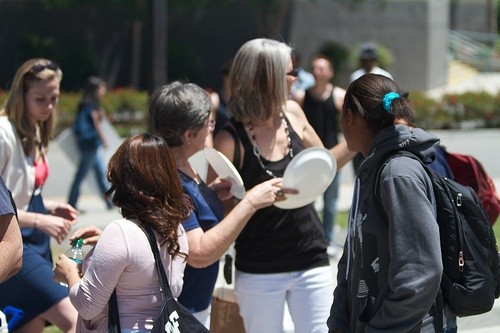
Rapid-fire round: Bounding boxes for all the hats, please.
[360,45,378,60]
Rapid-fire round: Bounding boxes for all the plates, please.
[203,147,246,199]
[272,146,337,209]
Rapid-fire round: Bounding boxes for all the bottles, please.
[54,237,83,287]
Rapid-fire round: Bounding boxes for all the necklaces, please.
[245,111,293,178]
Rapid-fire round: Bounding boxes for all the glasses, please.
[203,118,218,132]
[284,67,301,78]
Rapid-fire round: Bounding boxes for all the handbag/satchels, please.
[210,288,246,333]
[105,216,212,332]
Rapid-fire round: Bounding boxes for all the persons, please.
[0,58,79,333]
[351,45,394,83]
[207,38,360,333]
[54,133,196,333]
[327,73,457,333]
[68,76,114,212]
[149,82,299,333]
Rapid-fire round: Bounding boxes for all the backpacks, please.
[442,149,499,226]
[373,151,500,318]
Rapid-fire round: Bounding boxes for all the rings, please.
[277,190,283,196]
